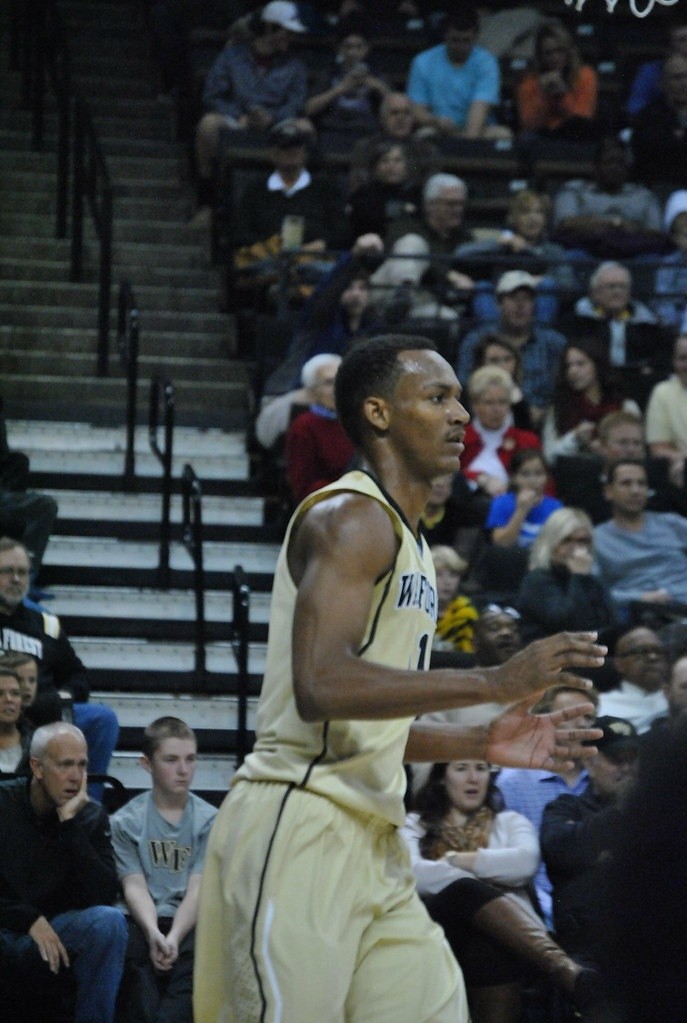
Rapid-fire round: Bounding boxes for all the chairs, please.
[223,144,595,348]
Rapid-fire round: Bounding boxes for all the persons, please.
[107,715,220,1023]
[0,412,130,1023]
[193,335,608,1021]
[154,0,687,1023]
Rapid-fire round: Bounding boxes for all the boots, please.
[473,891,622,1018]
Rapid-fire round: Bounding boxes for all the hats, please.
[269,117,320,156]
[581,714,650,751]
[493,269,540,296]
[259,3,307,34]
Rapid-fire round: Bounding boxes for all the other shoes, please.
[189,206,216,232]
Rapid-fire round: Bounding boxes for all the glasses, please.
[620,647,672,660]
[559,534,591,550]
